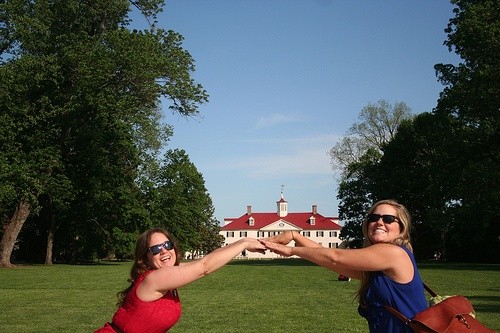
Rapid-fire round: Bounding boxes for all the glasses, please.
[148,241,174,255]
[368,213,399,223]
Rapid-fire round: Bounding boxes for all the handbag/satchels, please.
[409,295,497,333]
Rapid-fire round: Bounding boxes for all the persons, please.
[257,199,428,333]
[92,228,267,333]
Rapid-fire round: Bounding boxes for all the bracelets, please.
[291,230,294,240]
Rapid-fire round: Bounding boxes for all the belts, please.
[110,322,124,333]
